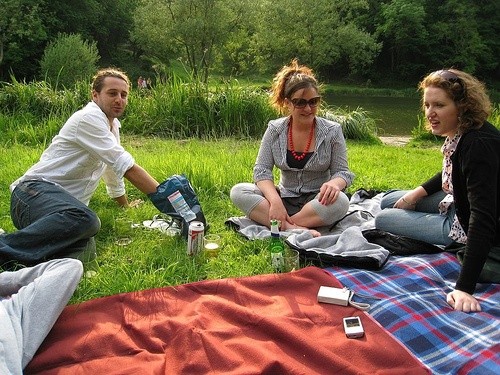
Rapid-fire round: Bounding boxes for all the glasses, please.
[288,96,321,107]
[434,70,457,83]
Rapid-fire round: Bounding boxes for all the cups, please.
[204,234,220,259]
[116,218,133,246]
[280,249,299,273]
[78,251,98,278]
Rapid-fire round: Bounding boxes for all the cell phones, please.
[342,316,364,339]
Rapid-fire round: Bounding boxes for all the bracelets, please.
[401,197,416,206]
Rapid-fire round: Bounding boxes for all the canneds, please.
[187,221,204,255]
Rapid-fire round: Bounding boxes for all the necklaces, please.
[289,117,316,160]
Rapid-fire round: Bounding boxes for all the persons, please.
[230,57,355,237]
[0,68,160,272]
[375,68,500,312]
[0,258,83,375]
[138,76,152,90]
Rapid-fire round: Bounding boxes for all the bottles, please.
[270,220,284,271]
